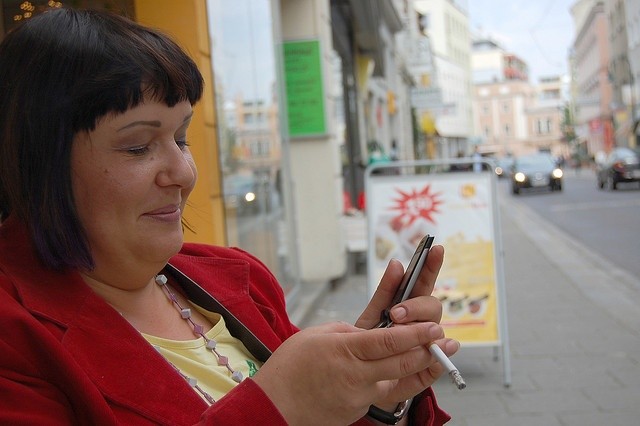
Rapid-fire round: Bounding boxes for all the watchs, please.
[368,397,414,425]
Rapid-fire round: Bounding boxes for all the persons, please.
[1,5,463,426]
[469,145,487,171]
[367,140,390,167]
[449,152,468,170]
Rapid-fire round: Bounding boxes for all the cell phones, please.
[369,234,433,328]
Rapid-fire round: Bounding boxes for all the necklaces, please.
[118,273,245,407]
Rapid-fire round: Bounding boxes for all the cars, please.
[510,152,562,193]
[597,147,639,188]
